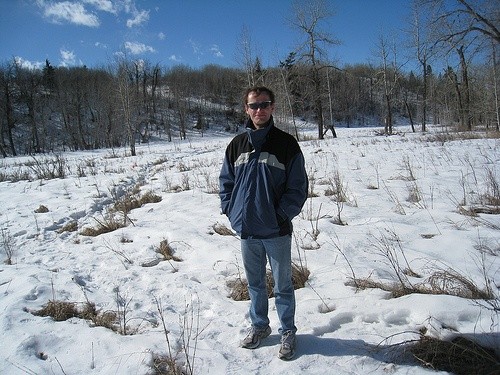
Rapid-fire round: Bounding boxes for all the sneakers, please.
[277,332,296,361]
[241,324,272,348]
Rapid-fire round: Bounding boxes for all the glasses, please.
[247,99,272,109]
